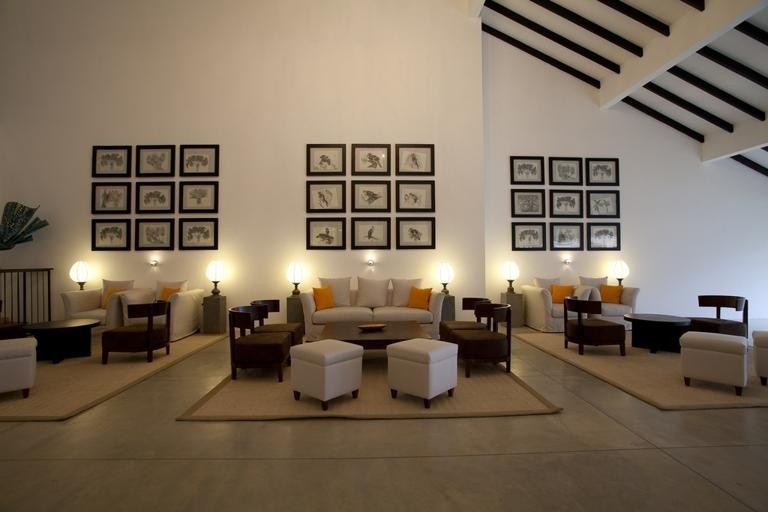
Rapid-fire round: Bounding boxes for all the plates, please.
[358,324,386,330]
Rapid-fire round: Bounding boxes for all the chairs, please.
[0,278,204,397]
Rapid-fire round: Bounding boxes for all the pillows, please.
[533,275,623,304]
[313,285,333,310]
[356,276,389,307]
[321,276,351,306]
[392,278,420,307]
[409,285,432,308]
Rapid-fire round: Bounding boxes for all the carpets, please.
[498,325,768,411]
[0,333,228,420]
[174,361,563,422]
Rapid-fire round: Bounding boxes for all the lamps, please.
[289,264,304,296]
[206,261,225,294]
[613,260,630,286]
[69,262,91,289]
[502,260,518,289]
[438,264,454,293]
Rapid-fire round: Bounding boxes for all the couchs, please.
[522,284,639,332]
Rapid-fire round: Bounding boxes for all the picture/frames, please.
[509,155,621,251]
[90,144,219,251]
[305,142,436,250]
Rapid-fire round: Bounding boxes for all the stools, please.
[386,338,458,409]
[679,329,768,396]
[289,338,365,411]
